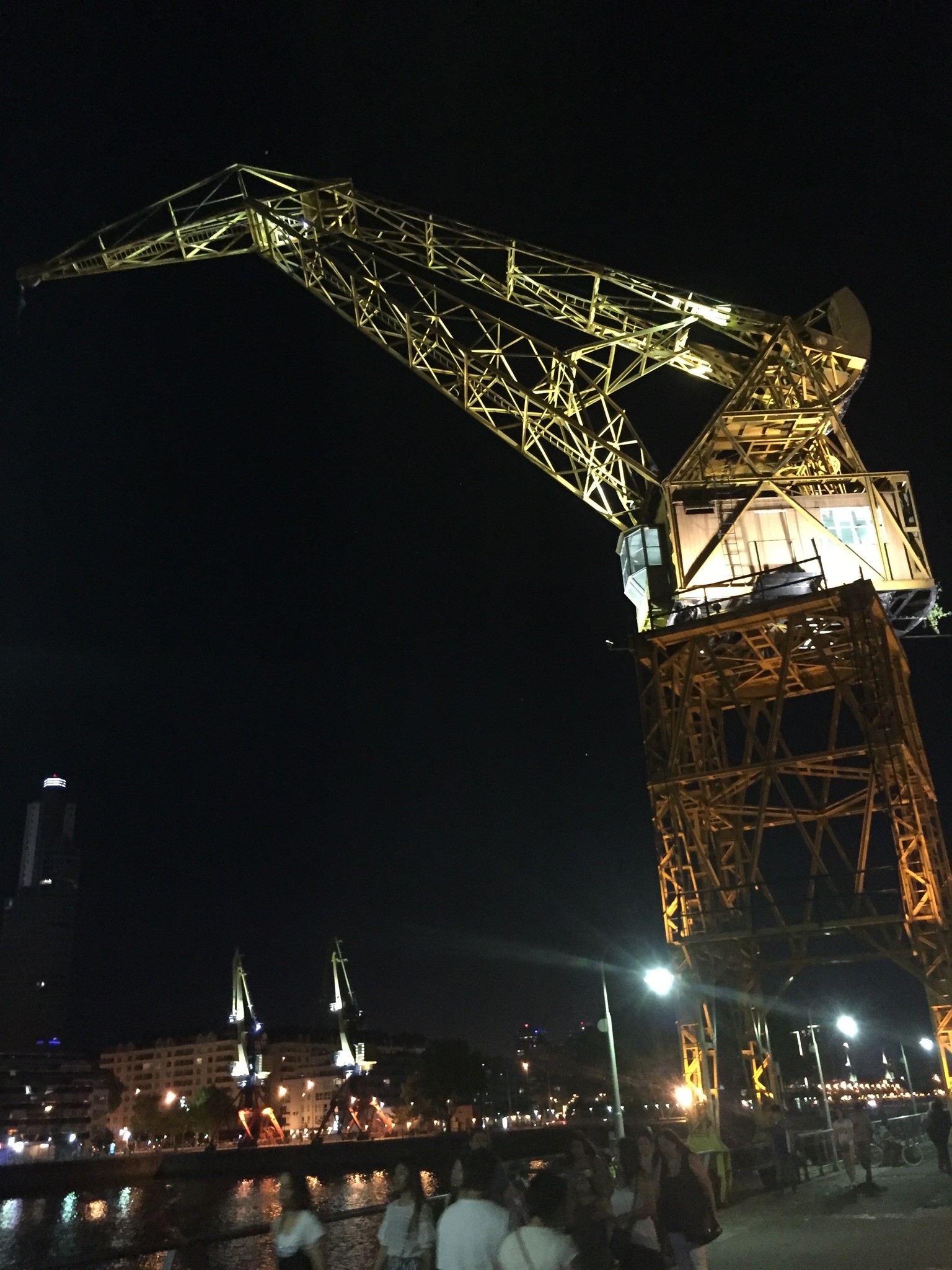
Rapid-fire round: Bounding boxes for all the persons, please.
[925,1098,952,1175]
[762,1105,799,1198]
[830,1103,874,1188]
[370,1128,720,1270]
[269,1169,326,1270]
[878,1118,903,1166]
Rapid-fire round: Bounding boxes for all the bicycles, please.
[865,1119,925,1168]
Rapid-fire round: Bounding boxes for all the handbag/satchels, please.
[690,1206,723,1245]
[610,1228,633,1259]
[396,1196,422,1270]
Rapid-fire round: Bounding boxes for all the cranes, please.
[13,158,952,1209]
